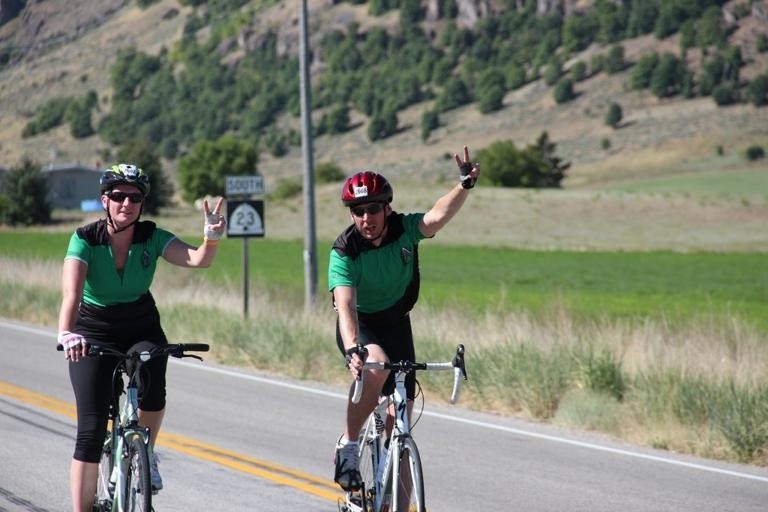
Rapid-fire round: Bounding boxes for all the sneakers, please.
[134,447,162,492]
[334,434,363,492]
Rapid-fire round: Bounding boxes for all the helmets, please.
[341,171,393,208]
[98,164,151,196]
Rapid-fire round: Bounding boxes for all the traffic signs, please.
[227,200,265,238]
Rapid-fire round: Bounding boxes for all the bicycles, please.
[57,340,210,512]
[336,343,470,511]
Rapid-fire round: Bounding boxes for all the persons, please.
[56,163,224,511]
[328,146,479,512]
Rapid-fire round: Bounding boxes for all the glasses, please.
[105,192,143,204]
[350,204,380,217]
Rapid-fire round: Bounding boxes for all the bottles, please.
[109,459,117,485]
[375,436,391,485]
